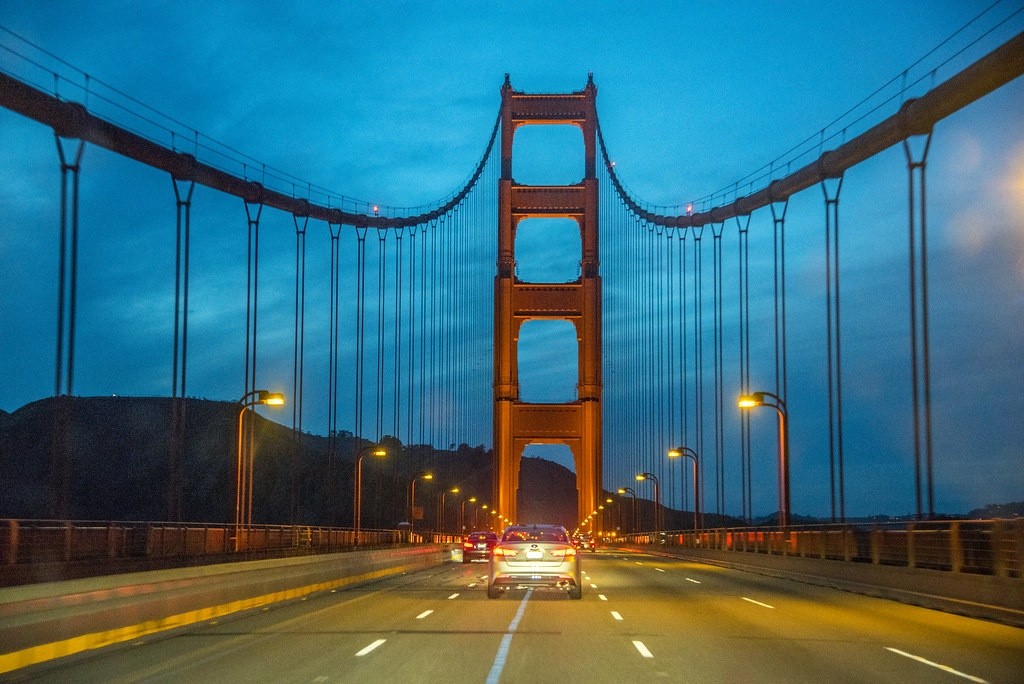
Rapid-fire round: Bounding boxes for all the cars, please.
[462,532,501,564]
[488,525,582,600]
[573,531,596,553]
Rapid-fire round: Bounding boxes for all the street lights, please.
[473,504,487,533]
[668,446,699,533]
[636,473,661,532]
[618,487,638,535]
[461,496,476,534]
[409,471,433,531]
[484,510,496,533]
[738,391,790,527]
[354,446,387,548]
[441,487,459,533]
[232,390,285,557]
[598,505,612,534]
[606,497,623,535]
